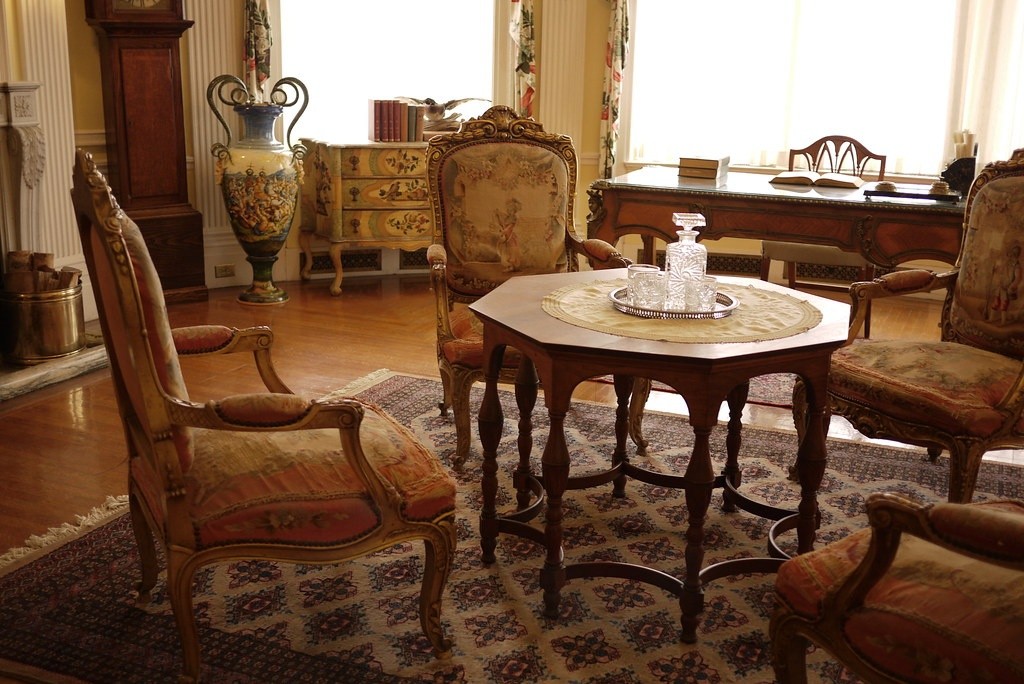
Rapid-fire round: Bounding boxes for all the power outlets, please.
[214,263,236,278]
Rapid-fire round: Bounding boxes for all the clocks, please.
[83,0,211,306]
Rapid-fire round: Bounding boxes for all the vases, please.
[205,74,308,304]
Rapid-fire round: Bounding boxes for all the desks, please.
[583,164,968,342]
[468,266,854,643]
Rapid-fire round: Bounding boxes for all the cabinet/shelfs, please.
[297,137,435,299]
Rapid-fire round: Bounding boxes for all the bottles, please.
[664,212,707,310]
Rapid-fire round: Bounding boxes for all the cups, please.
[631,272,668,312]
[627,264,660,304]
[685,275,717,313]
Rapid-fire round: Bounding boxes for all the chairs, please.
[764,495,1024,684]
[761,135,886,340]
[428,106,650,468]
[64,152,458,684]
[785,149,1024,503]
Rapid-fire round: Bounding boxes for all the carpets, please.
[0,369,1024,684]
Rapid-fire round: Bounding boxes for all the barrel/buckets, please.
[0,279,88,369]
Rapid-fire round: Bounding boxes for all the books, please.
[678,156,730,179]
[368,99,425,142]
[769,171,864,189]
